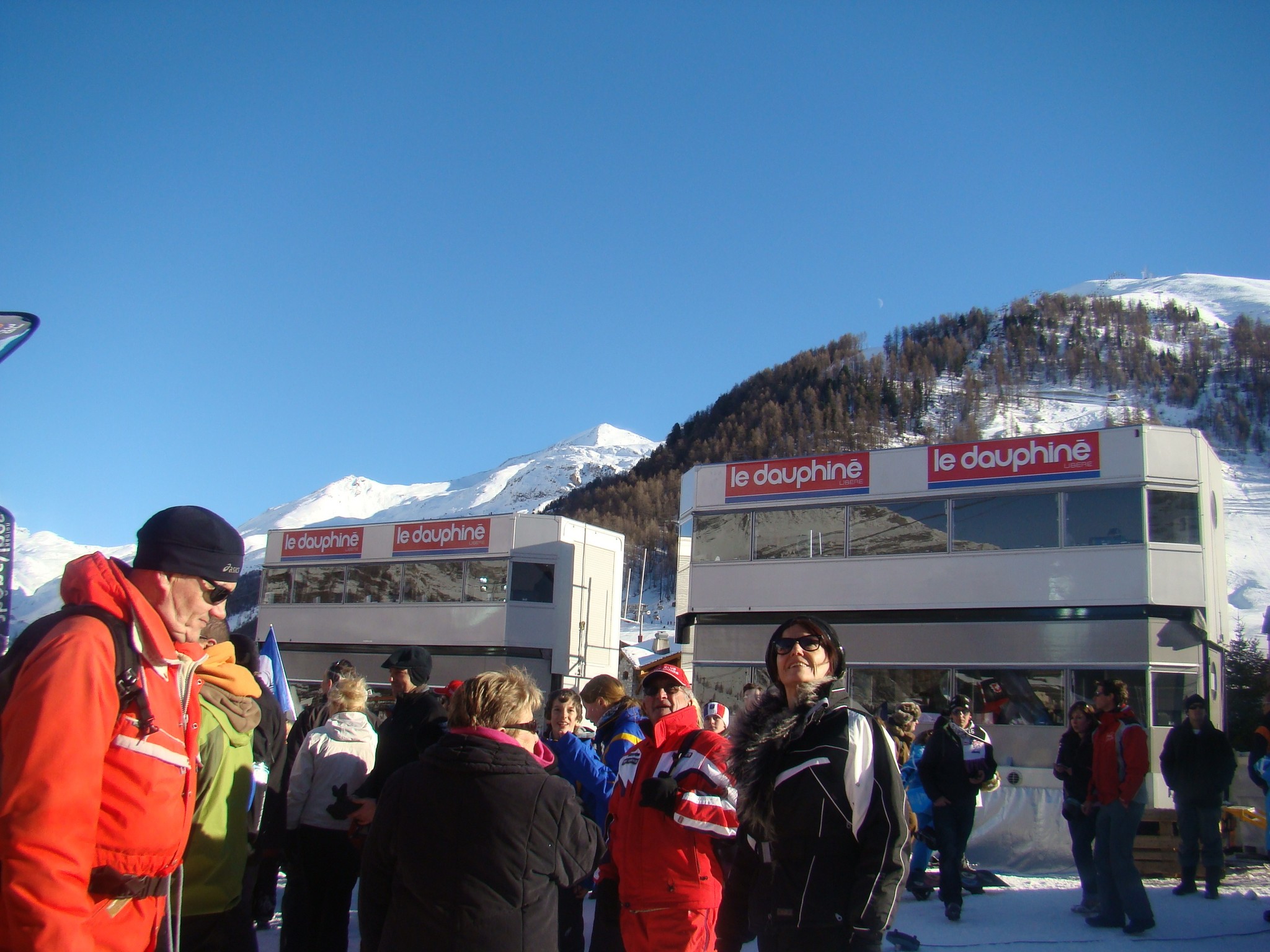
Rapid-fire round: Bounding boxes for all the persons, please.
[163,617,998,952]
[1247,694,1270,924]
[0,505,244,951]
[1053,679,1156,935]
[1159,694,1238,898]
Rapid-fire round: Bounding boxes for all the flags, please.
[258,623,294,712]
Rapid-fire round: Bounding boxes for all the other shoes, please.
[1172,880,1197,895]
[944,902,959,920]
[1070,904,1086,914]
[902,878,930,901]
[256,918,270,929]
[1123,916,1154,934]
[1204,887,1219,899]
[1085,914,1123,927]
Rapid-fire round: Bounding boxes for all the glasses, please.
[952,708,970,716]
[643,682,683,696]
[773,633,829,655]
[440,698,448,705]
[502,719,536,735]
[201,576,233,606]
[1095,691,1104,696]
[1189,704,1205,710]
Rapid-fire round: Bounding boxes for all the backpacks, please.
[0,601,157,746]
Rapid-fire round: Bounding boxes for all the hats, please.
[133,505,244,583]
[950,695,971,712]
[434,679,464,698]
[894,701,921,726]
[642,664,692,689]
[380,646,432,687]
[704,701,729,728]
[1185,694,1206,706]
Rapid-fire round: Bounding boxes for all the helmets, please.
[765,615,846,675]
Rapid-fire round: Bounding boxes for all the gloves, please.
[639,770,678,821]
